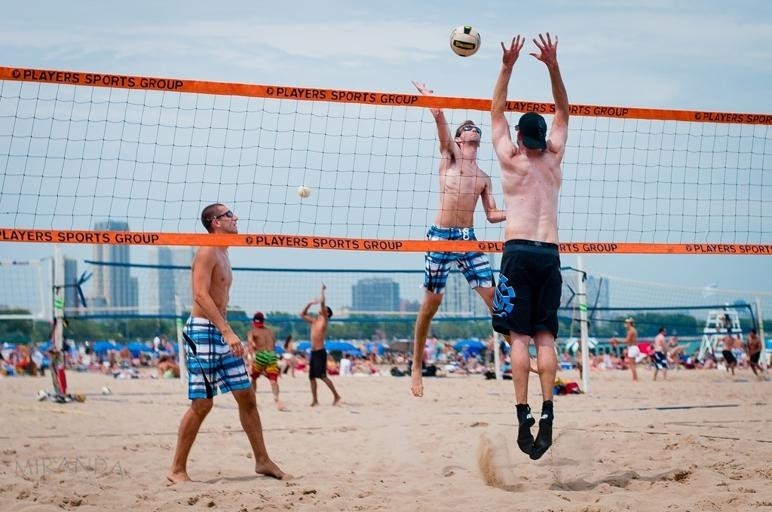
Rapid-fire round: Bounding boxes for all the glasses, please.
[458,126,482,137]
[215,210,234,219]
[514,124,520,131]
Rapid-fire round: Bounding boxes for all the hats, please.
[517,111,547,150]
[251,312,265,328]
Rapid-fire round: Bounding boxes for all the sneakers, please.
[515,402,535,454]
[530,399,554,460]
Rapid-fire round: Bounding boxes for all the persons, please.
[165,203,294,484]
[490,32,571,460]
[411,79,540,398]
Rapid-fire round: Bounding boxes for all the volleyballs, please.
[451,25,481,56]
[297,186,311,198]
